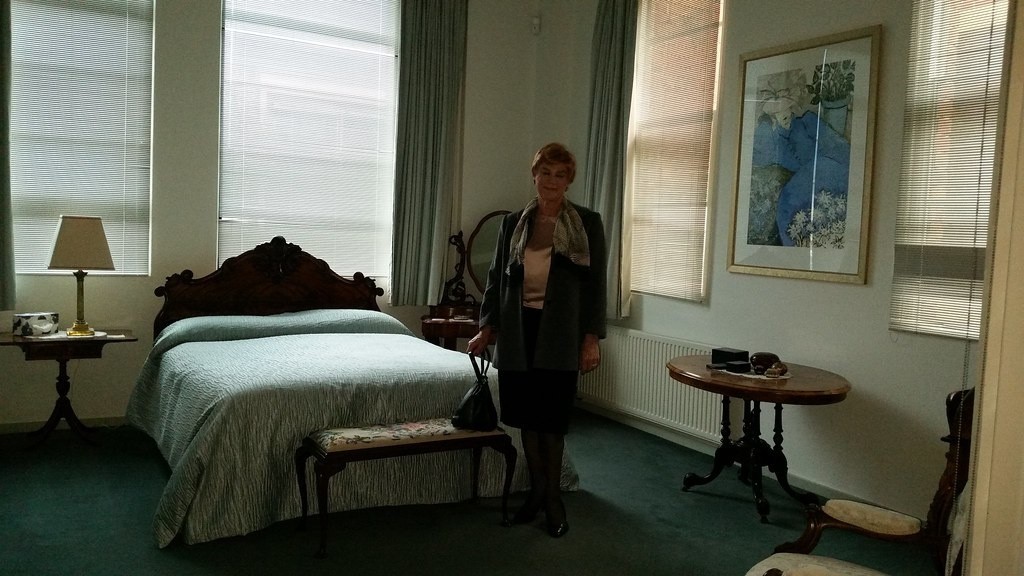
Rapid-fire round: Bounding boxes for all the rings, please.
[592,364,597,366]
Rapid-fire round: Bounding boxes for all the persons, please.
[464,142,608,536]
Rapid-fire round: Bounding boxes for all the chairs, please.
[736,383,978,576]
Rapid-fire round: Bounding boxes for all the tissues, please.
[13,312,60,338]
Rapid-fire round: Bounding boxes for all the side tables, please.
[419,304,501,360]
[0,327,138,446]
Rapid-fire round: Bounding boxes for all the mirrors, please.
[467,209,516,300]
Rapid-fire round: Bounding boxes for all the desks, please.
[668,353,854,522]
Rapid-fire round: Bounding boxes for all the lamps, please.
[46,213,116,337]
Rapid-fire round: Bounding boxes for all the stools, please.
[293,418,518,544]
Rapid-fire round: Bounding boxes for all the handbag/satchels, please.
[452,346,499,433]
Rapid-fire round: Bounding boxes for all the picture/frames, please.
[724,22,884,288]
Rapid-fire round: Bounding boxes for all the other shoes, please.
[513,501,570,537]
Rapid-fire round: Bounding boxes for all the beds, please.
[153,308,506,551]
